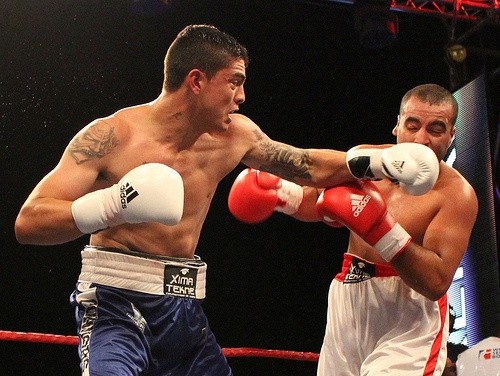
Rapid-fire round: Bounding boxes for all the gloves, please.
[315,180,412,263]
[228,167,305,222]
[345,142,439,197]
[71,162,185,233]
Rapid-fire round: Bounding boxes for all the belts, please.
[78,249,207,299]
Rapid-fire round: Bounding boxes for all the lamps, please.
[353,2,399,48]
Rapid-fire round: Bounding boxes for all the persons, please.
[443,41,480,92]
[15,25,439,376]
[227,84,478,376]
